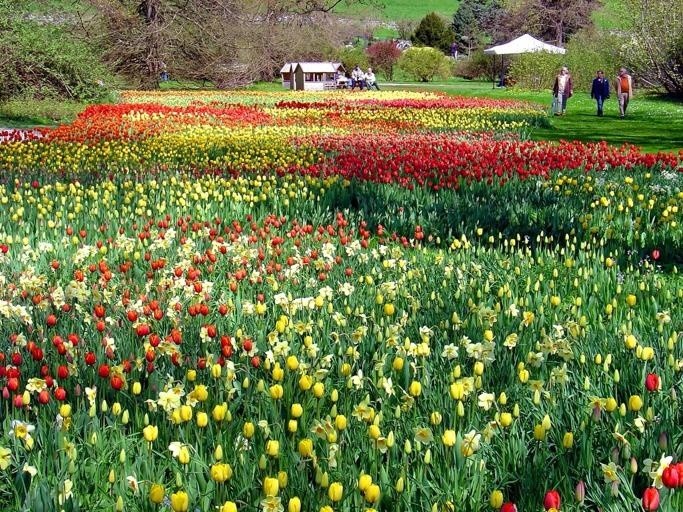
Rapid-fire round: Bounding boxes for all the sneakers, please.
[597,111,604,117]
[620,114,626,119]
[554,111,567,116]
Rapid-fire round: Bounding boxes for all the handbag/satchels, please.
[552,94,560,112]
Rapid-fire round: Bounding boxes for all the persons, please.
[335,75,347,88]
[450,42,458,61]
[551,66,573,116]
[350,65,364,91]
[364,67,380,91]
[612,67,633,119]
[590,70,610,117]
